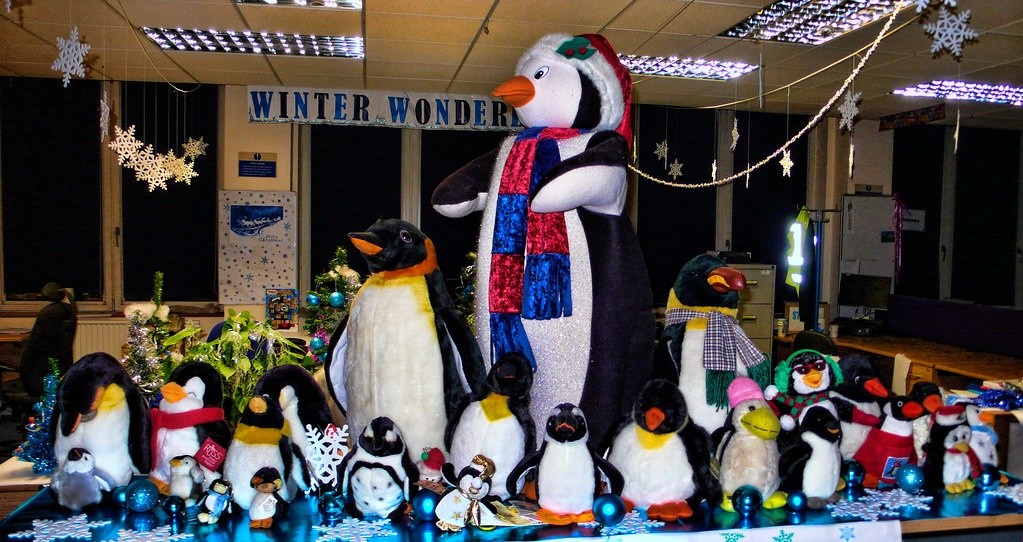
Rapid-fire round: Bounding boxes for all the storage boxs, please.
[726,263,778,362]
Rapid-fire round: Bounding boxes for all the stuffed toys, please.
[432,32,656,459]
[653,254,770,439]
[49,348,999,532]
[324,218,488,463]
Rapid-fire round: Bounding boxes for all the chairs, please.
[0,300,79,458]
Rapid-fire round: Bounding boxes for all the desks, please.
[0,326,32,373]
[775,334,1023,395]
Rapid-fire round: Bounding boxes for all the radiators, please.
[73,321,132,365]
